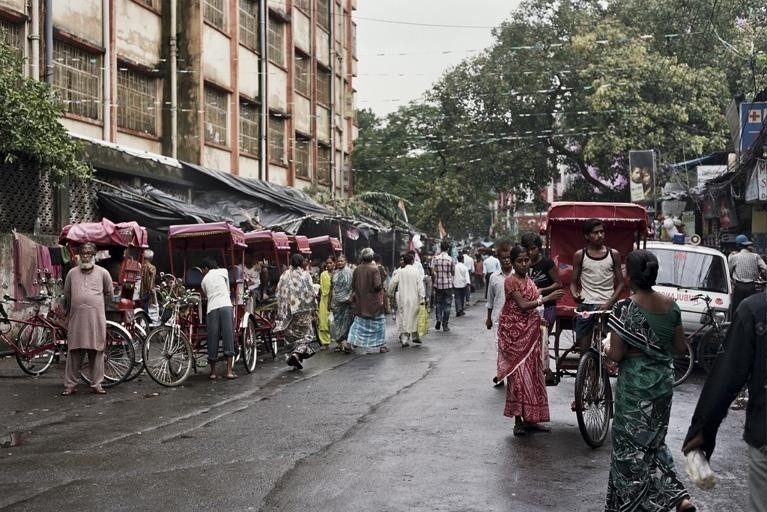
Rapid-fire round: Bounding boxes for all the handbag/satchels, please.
[141,303,162,327]
[417,303,429,336]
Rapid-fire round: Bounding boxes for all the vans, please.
[633,238,731,337]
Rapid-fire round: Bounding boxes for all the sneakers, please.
[399,331,422,347]
[287,352,303,369]
[514,423,552,437]
[456,300,470,316]
[571,398,584,411]
[435,320,449,332]
[603,357,619,376]
[493,376,504,386]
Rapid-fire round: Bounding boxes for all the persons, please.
[200,258,241,380]
[569,219,625,413]
[517,230,561,382]
[727,235,767,318]
[493,244,563,439]
[485,247,516,388]
[630,164,652,200]
[245,227,500,369]
[604,251,695,511]
[681,286,767,511]
[62,242,115,397]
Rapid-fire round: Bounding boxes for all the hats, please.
[735,234,753,246]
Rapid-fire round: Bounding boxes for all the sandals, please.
[319,339,357,353]
[209,372,238,379]
[62,383,106,395]
[676,499,696,512]
[380,345,390,353]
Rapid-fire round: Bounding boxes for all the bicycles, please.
[654,296,739,387]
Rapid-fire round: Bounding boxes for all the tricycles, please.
[0,217,344,389]
[542,198,649,454]
